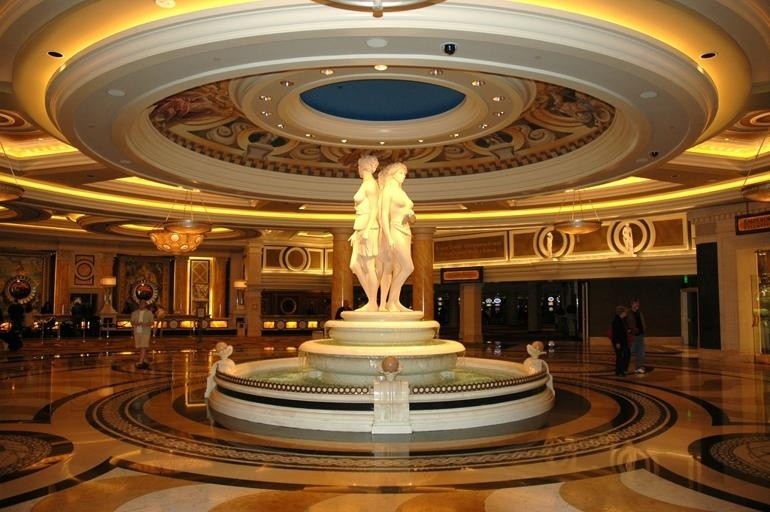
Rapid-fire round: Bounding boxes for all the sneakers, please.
[135,362,147,368]
[634,366,646,373]
[616,370,628,376]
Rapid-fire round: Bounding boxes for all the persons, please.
[346,155,415,313]
[0,296,86,340]
[152,306,167,337]
[130,299,154,368]
[336,300,351,320]
[626,296,647,374]
[482,307,490,332]
[611,305,632,376]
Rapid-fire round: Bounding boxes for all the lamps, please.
[553,188,603,235]
[147,186,212,256]
[0,141,25,202]
[97,276,118,314]
[739,127,770,202]
[233,281,247,314]
